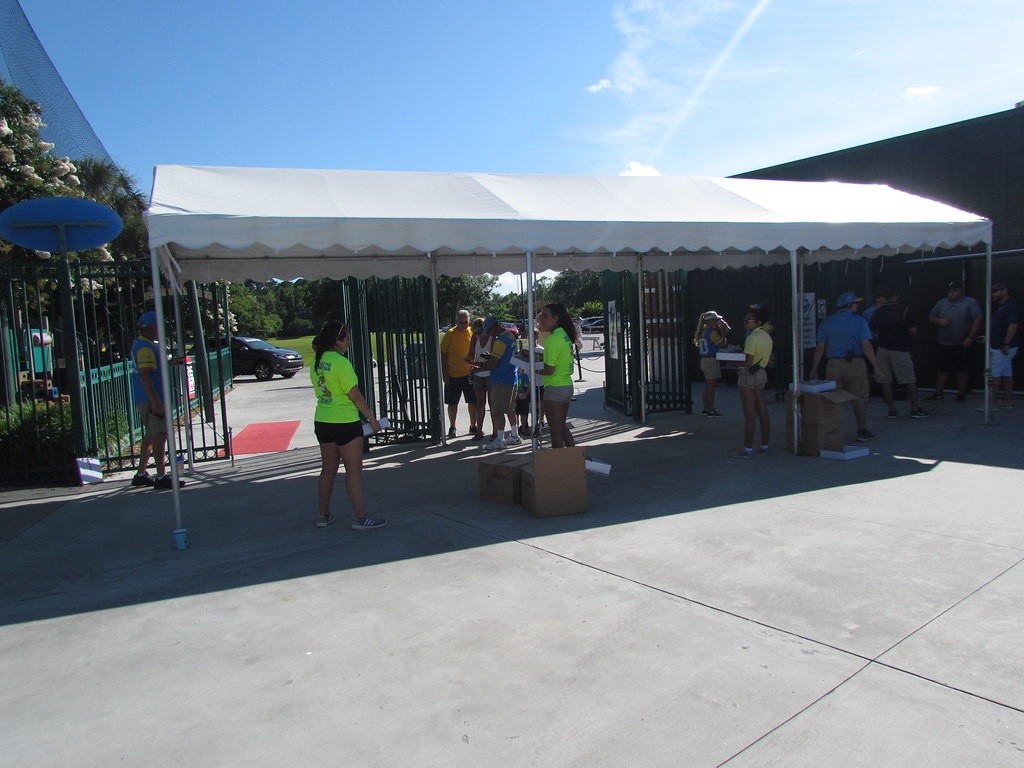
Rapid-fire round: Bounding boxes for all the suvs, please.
[187,337,304,381]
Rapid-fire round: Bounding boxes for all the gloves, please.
[152,400,164,416]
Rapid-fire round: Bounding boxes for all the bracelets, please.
[968,336,975,339]
[366,416,375,421]
[1003,343,1009,346]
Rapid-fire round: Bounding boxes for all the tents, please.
[143,164,993,530]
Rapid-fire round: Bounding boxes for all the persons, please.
[976,281,1024,412]
[860,283,930,419]
[929,279,982,403]
[131,312,185,490]
[693,311,728,418]
[440,304,584,450]
[310,320,388,529]
[809,291,882,441]
[728,309,776,459]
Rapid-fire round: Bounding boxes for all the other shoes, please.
[448,421,529,450]
[924,394,945,401]
[952,394,967,403]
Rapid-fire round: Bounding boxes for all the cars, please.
[578,317,604,331]
[499,322,539,339]
[442,325,452,332]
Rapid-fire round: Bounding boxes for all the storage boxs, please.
[716,352,747,361]
[478,447,586,517]
[786,380,870,460]
[22,380,58,398]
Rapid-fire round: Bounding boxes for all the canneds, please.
[718,319,730,332]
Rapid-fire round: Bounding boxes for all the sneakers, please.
[131,472,154,486]
[976,403,1000,412]
[351,512,388,529]
[702,409,724,417]
[760,444,771,455]
[315,515,335,527]
[154,474,185,489]
[998,404,1013,410]
[729,447,753,458]
[887,408,897,419]
[856,429,875,439]
[910,406,931,417]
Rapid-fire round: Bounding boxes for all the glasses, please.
[991,289,998,293]
[744,319,758,323]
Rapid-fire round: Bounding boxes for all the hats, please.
[482,315,498,333]
[703,310,723,321]
[836,291,864,308]
[138,311,157,329]
[991,282,1007,290]
[944,278,963,289]
